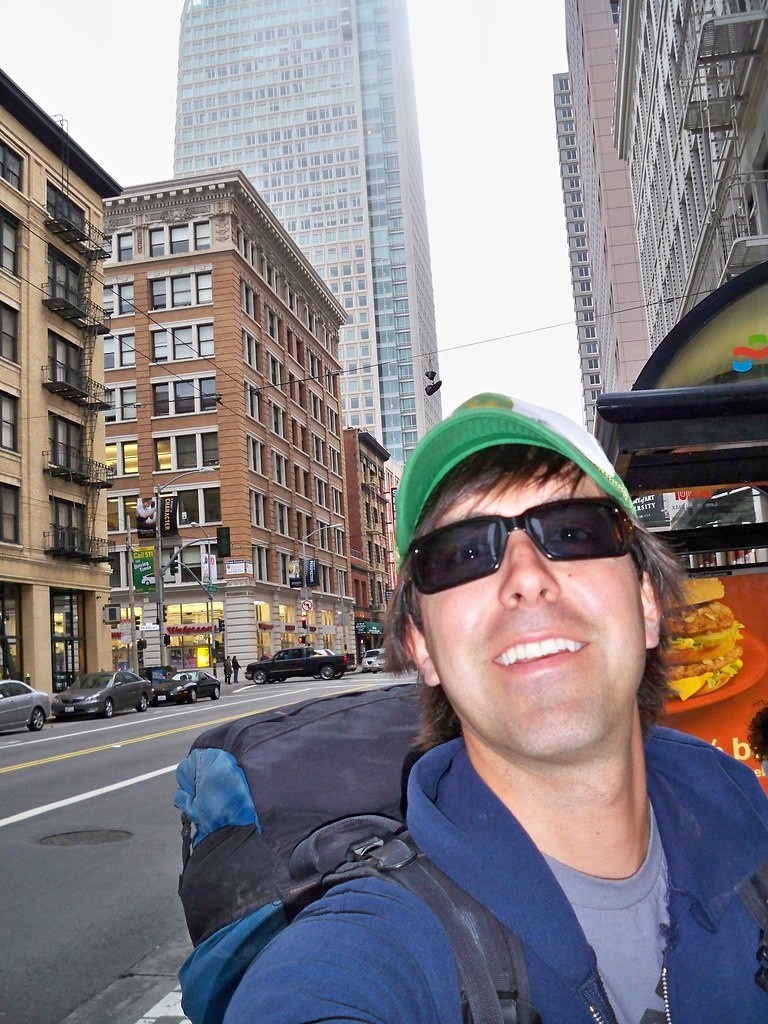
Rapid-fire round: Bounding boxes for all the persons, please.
[137,497,156,525]
[224,659,228,683]
[222,393,768,1024]
[226,656,232,684]
[259,652,269,661]
[232,656,241,683]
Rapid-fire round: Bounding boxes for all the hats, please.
[391,394,634,570]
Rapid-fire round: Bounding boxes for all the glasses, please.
[408,497,631,594]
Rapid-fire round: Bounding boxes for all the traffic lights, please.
[163,605,167,622]
[135,616,140,630]
[169,552,179,576]
[219,619,225,633]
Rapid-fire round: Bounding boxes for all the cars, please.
[149,670,221,704]
[0,679,51,731]
[361,648,386,674]
[50,669,153,717]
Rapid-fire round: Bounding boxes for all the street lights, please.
[302,522,343,646]
[155,465,214,667]
[190,522,217,680]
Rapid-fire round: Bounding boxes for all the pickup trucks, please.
[243,646,348,684]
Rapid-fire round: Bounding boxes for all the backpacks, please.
[176,681,530,1023]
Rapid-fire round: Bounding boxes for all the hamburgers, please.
[662,578,744,695]
